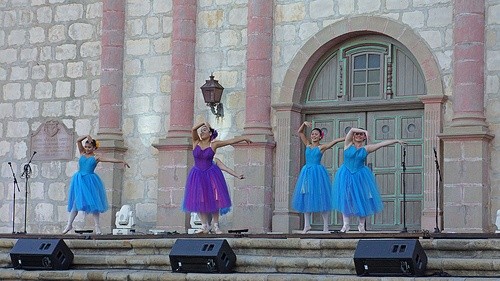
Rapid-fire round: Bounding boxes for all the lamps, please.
[201,71,224,118]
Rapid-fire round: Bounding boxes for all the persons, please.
[330,128,404,232]
[289,122,355,234]
[62,135,130,234]
[181,121,251,232]
[209,156,245,235]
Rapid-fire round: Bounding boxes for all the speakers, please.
[9,238,74,270]
[353,240,428,276]
[169,239,236,274]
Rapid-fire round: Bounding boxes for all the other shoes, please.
[304,226,311,233]
[341,225,350,232]
[214,230,223,234]
[324,227,328,232]
[358,225,365,232]
[195,228,209,234]
[62,226,73,234]
[96,228,101,234]
[207,224,212,230]
